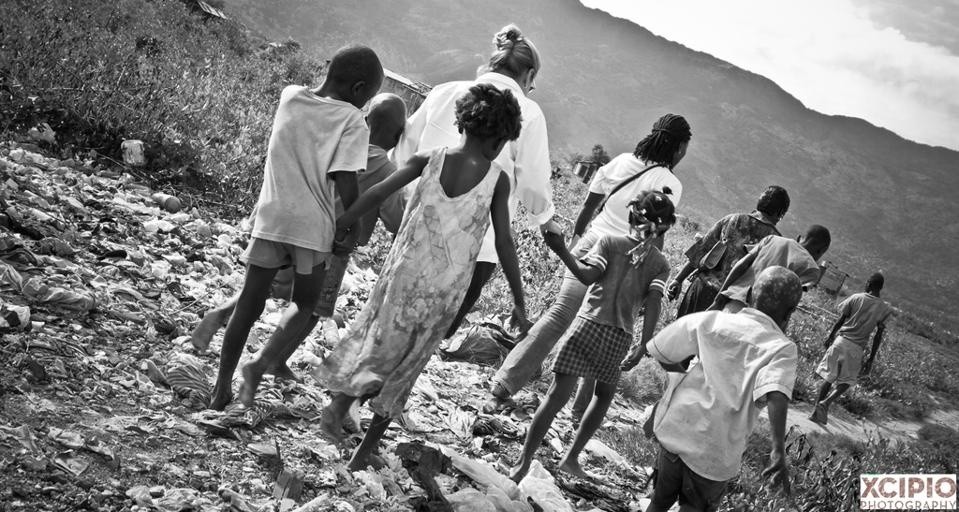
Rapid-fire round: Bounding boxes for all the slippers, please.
[816,402,828,425]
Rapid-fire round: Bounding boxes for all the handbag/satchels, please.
[699,241,727,271]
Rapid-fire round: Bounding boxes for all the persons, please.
[511,191,675,480]
[323,82,531,471]
[811,270,893,422]
[669,185,790,319]
[191,95,409,383]
[392,25,554,338]
[640,266,802,512]
[205,42,383,410]
[491,113,686,401]
[705,224,830,333]
